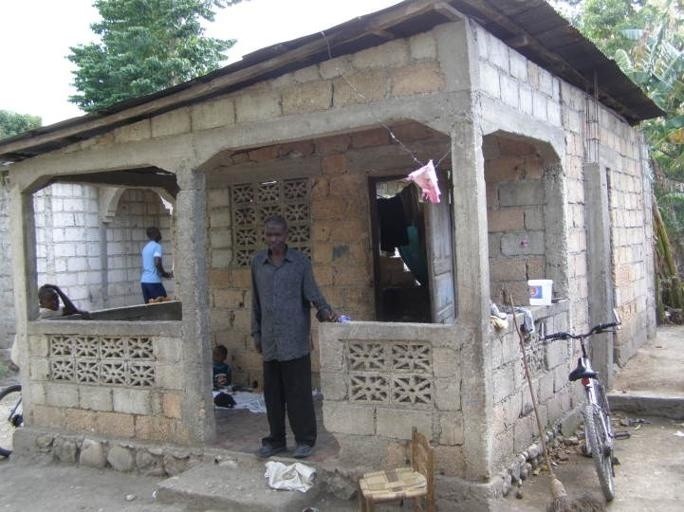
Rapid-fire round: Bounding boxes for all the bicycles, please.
[1,384,24,457]
[542,322,632,501]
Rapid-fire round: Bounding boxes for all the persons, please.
[213,344,233,391]
[140,226,173,303]
[250,215,338,459]
[10,284,87,367]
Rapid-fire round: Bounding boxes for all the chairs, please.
[356,425,434,512]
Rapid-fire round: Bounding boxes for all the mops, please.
[506,290,607,512]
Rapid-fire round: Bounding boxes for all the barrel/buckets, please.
[526,280,553,305]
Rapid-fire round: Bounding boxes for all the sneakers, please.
[292,443,314,458]
[255,440,286,458]
